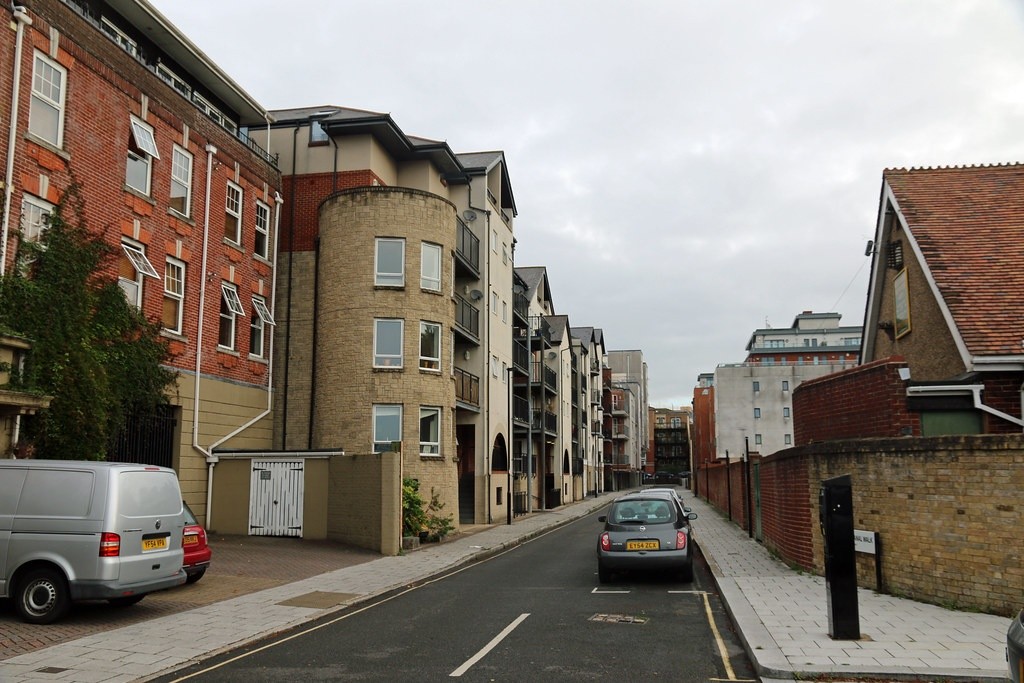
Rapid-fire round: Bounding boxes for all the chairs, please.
[619,508,634,519]
[654,505,669,519]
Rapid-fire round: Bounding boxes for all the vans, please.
[0,458,188,624]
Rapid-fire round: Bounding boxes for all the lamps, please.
[463,350,469,359]
[464,285,468,293]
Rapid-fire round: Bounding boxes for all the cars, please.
[183,500,213,583]
[596,494,698,582]
[641,488,693,517]
